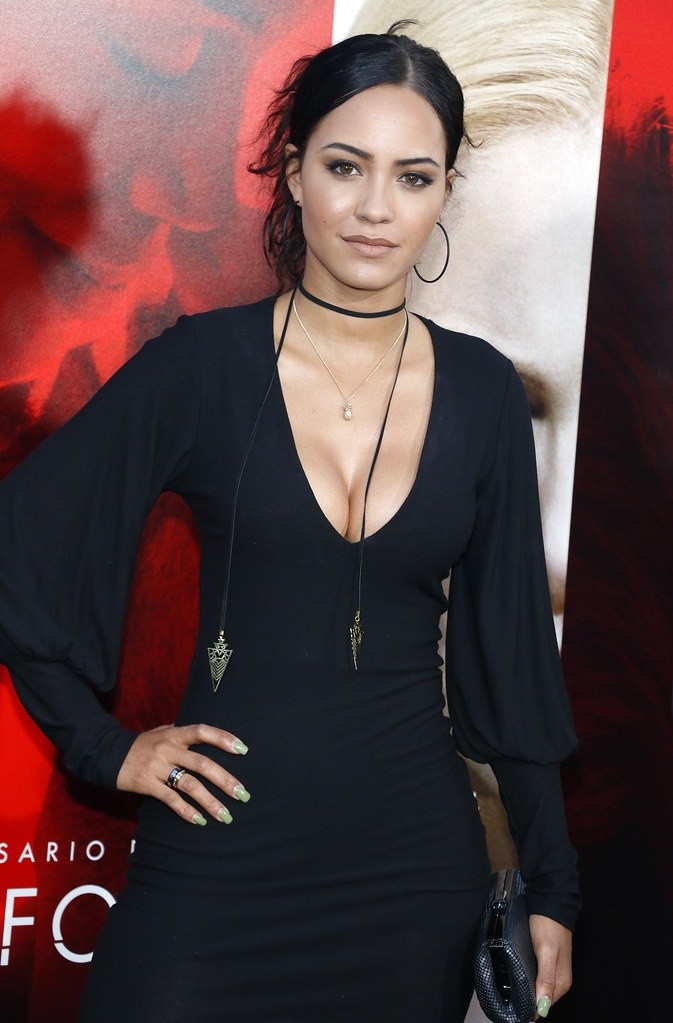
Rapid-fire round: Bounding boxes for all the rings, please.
[165,767,185,787]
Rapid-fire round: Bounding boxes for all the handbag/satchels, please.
[474,868,538,1023]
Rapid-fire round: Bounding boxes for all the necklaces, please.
[205,265,410,693]
[292,300,409,421]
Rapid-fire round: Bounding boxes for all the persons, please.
[1,1,314,1022]
[344,0,611,872]
[1,21,577,1023]
